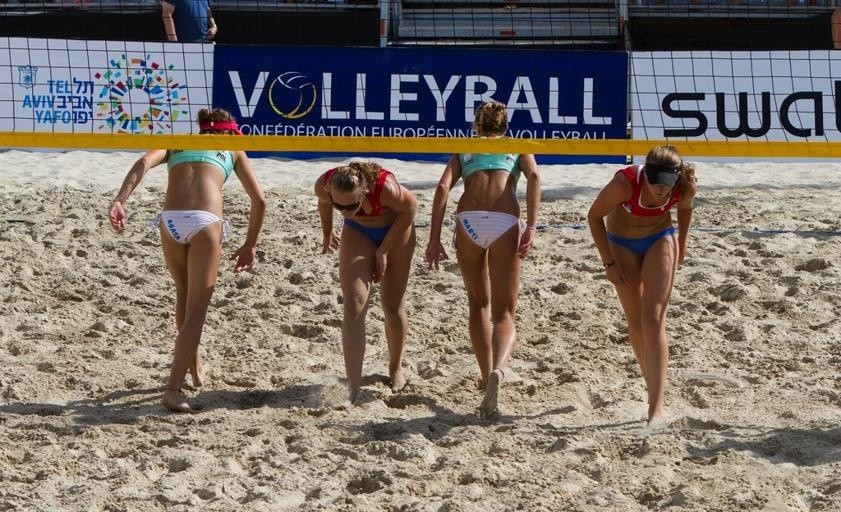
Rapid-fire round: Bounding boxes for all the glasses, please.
[332,202,360,213]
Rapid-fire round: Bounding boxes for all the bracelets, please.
[602,258,615,267]
[527,226,536,230]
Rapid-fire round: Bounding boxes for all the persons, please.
[424,100,543,424]
[586,142,698,430]
[312,160,417,405]
[106,106,268,414]
[161,1,219,43]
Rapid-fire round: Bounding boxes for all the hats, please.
[646,162,679,186]
[199,122,243,136]
